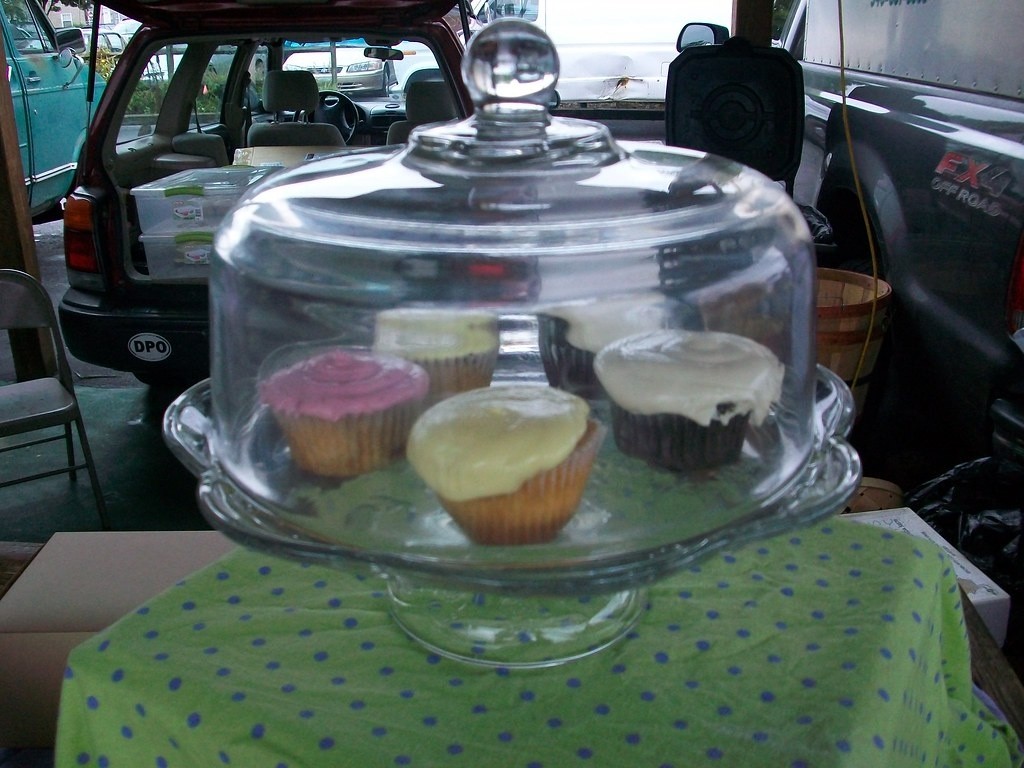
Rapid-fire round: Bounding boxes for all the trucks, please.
[389,0,736,113]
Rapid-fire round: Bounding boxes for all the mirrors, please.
[54,29,86,54]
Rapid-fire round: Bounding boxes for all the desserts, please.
[257,350,429,474]
[590,327,787,474]
[372,306,500,403]
[531,287,675,401]
[405,383,609,546]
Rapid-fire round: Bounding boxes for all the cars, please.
[282,39,397,97]
[11,16,269,105]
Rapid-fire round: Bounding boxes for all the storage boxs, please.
[139,214,223,279]
[130,166,283,235]
[837,507,1009,648]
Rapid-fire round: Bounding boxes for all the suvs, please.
[677,0,1024,505]
[0,0,107,219]
[59,0,560,386]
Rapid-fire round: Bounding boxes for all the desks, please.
[69,401,942,768]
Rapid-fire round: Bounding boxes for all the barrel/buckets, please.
[699,267,892,417]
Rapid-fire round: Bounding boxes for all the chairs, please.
[0,268,114,531]
[246,69,346,145]
[386,81,457,144]
